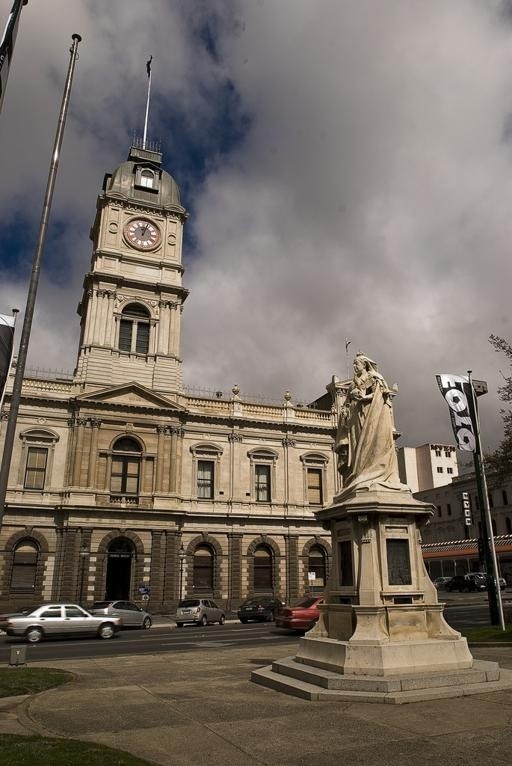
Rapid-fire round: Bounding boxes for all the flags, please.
[146,57,152,78]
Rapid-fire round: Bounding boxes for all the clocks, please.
[122,215,164,251]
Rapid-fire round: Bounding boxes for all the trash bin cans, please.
[10,645,27,664]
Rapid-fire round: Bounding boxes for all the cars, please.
[0,600,152,643]
[433,573,507,592]
[237,596,285,624]
[175,599,225,627]
[275,597,325,630]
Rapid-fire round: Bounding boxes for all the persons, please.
[331,355,401,496]
